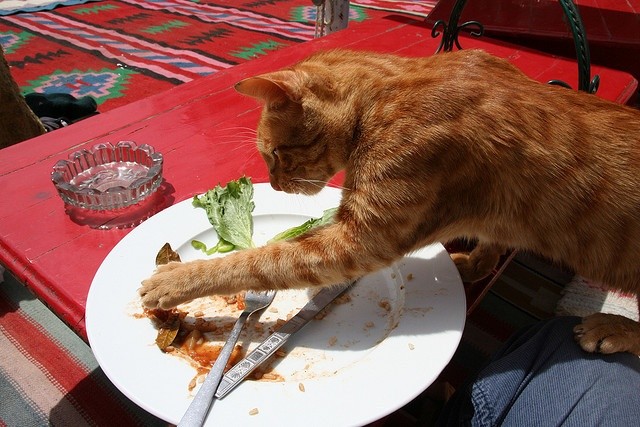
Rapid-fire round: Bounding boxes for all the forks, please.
[177,290,277,427]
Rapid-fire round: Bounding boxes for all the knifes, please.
[214,275,364,400]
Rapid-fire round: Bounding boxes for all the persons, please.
[470,316,640,426]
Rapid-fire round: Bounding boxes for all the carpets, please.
[380,239,605,424]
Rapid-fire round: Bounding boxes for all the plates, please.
[85,182,466,427]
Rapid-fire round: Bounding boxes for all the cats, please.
[137,45,640,359]
